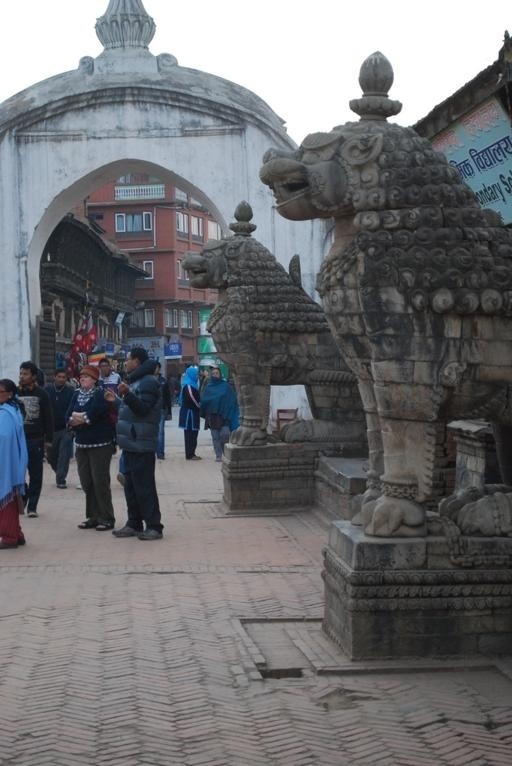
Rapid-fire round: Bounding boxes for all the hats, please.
[79,365,100,379]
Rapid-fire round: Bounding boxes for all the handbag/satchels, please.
[207,413,223,428]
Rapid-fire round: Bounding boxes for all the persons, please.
[96,358,238,462]
[14,360,54,518]
[107,346,163,542]
[45,370,76,489]
[0,377,29,550]
[66,365,116,530]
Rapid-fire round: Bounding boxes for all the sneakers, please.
[57,484,66,488]
[79,519,114,530]
[187,456,201,460]
[27,507,36,516]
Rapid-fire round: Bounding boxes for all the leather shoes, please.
[0,540,25,549]
[112,526,162,539]
